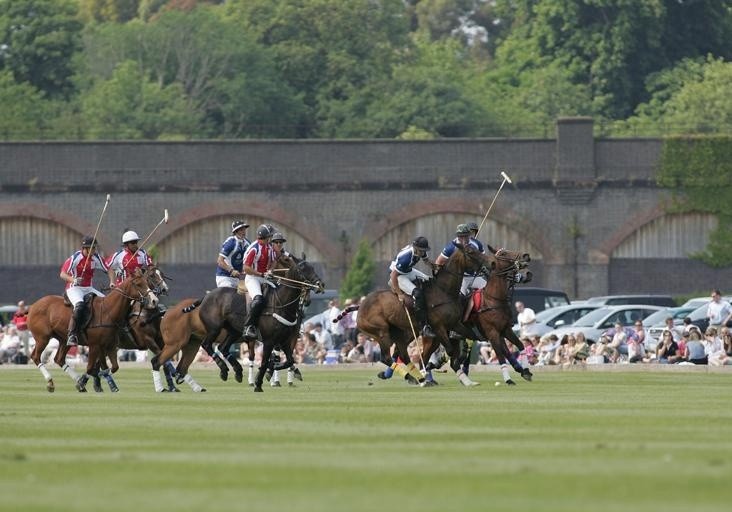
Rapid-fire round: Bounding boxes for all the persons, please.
[387,236,438,339]
[243,223,275,339]
[270,232,291,264]
[1,297,381,363]
[105,231,154,288]
[465,221,486,257]
[479,291,731,368]
[433,224,480,339]
[215,220,251,289]
[61,236,115,358]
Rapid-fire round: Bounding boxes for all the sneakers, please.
[423,326,434,337]
[245,325,258,339]
[68,334,76,344]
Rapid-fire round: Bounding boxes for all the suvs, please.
[509,285,732,344]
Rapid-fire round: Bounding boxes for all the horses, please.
[273,285,312,387]
[15,265,160,395]
[93,262,179,393]
[180,252,326,393]
[332,241,497,389]
[458,266,534,378]
[139,248,296,393]
[419,243,531,386]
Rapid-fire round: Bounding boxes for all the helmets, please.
[457,223,479,233]
[122,231,142,242]
[413,236,431,252]
[231,220,249,231]
[81,235,99,248]
[257,224,286,242]
